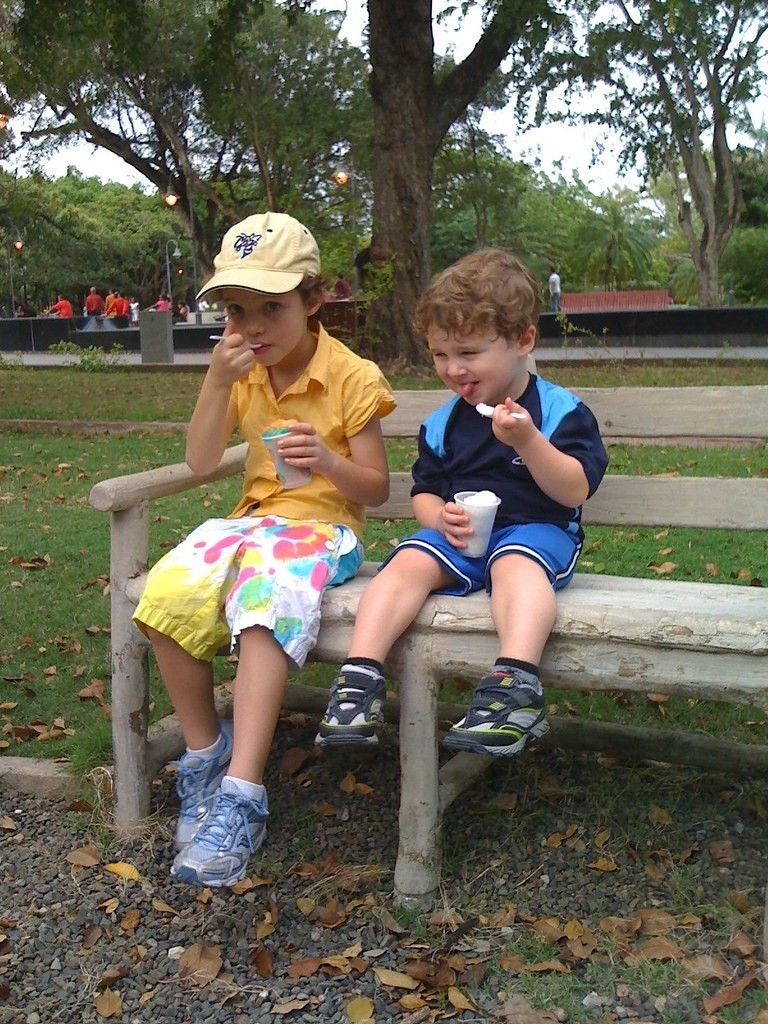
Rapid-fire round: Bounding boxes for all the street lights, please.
[332,144,361,357]
[6,224,24,318]
[165,167,202,325]
[165,239,183,309]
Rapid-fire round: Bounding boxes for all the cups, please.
[261,429,311,489]
[454,492,501,558]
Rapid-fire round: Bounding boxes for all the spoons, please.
[209,335,262,350]
[476,405,527,420]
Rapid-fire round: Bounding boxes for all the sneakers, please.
[313,665,386,750]
[170,779,270,888]
[442,664,551,759]
[166,719,234,851]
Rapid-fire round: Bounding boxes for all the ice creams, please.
[263,418,312,487]
[461,490,497,556]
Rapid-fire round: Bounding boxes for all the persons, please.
[547,266,562,313]
[16,306,25,318]
[133,213,397,889]
[83,287,141,328]
[45,294,74,318]
[333,272,352,301]
[314,251,609,757]
[144,289,210,322]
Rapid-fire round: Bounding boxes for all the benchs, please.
[88,386,768,916]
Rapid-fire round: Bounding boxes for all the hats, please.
[195,212,320,301]
